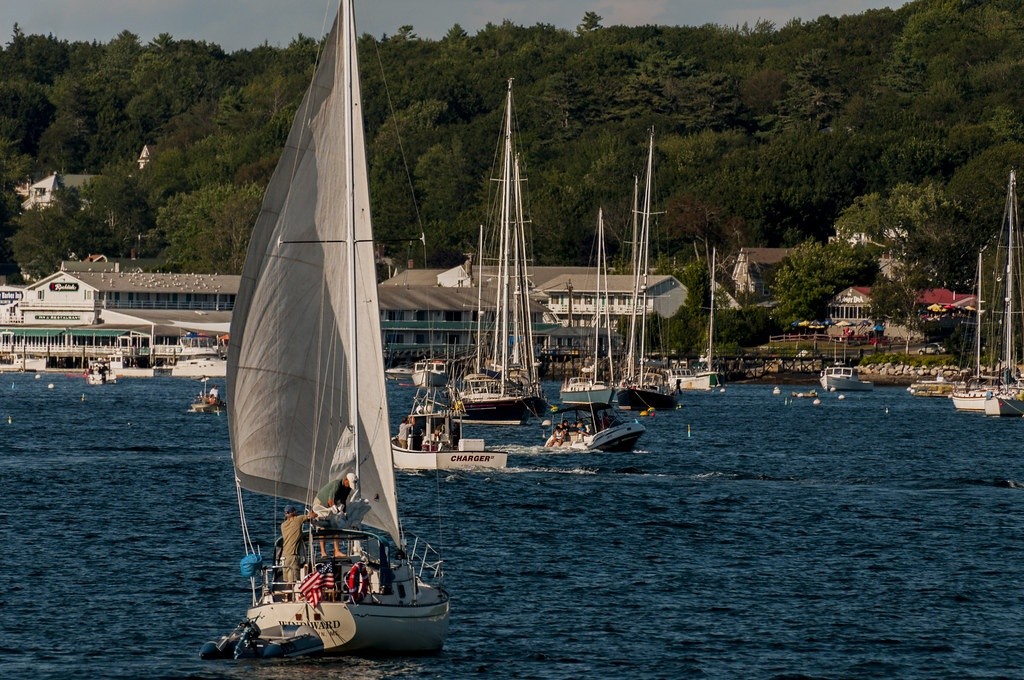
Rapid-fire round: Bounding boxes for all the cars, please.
[917,342,947,356]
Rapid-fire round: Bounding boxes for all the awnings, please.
[0,328,65,338]
[65,328,130,338]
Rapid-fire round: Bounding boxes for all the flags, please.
[298,562,336,607]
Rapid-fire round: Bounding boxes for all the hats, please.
[347,472,358,489]
[284,505,298,514]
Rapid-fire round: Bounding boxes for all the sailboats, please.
[200,1,451,661]
[948,166,1024,415]
[392,77,726,470]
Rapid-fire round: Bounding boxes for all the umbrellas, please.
[926,303,976,313]
[790,317,884,337]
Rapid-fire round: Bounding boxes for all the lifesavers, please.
[348,562,369,602]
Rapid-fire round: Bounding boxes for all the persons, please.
[312,472,357,557]
[398,415,460,450]
[126,356,134,367]
[281,505,319,601]
[591,409,600,433]
[87,363,108,373]
[601,412,610,428]
[618,375,676,397]
[545,420,590,447]
[208,384,218,403]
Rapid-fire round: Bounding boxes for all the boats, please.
[172,329,229,376]
[539,387,646,451]
[190,376,226,413]
[907,377,967,397]
[83,349,115,384]
[820,337,874,391]
